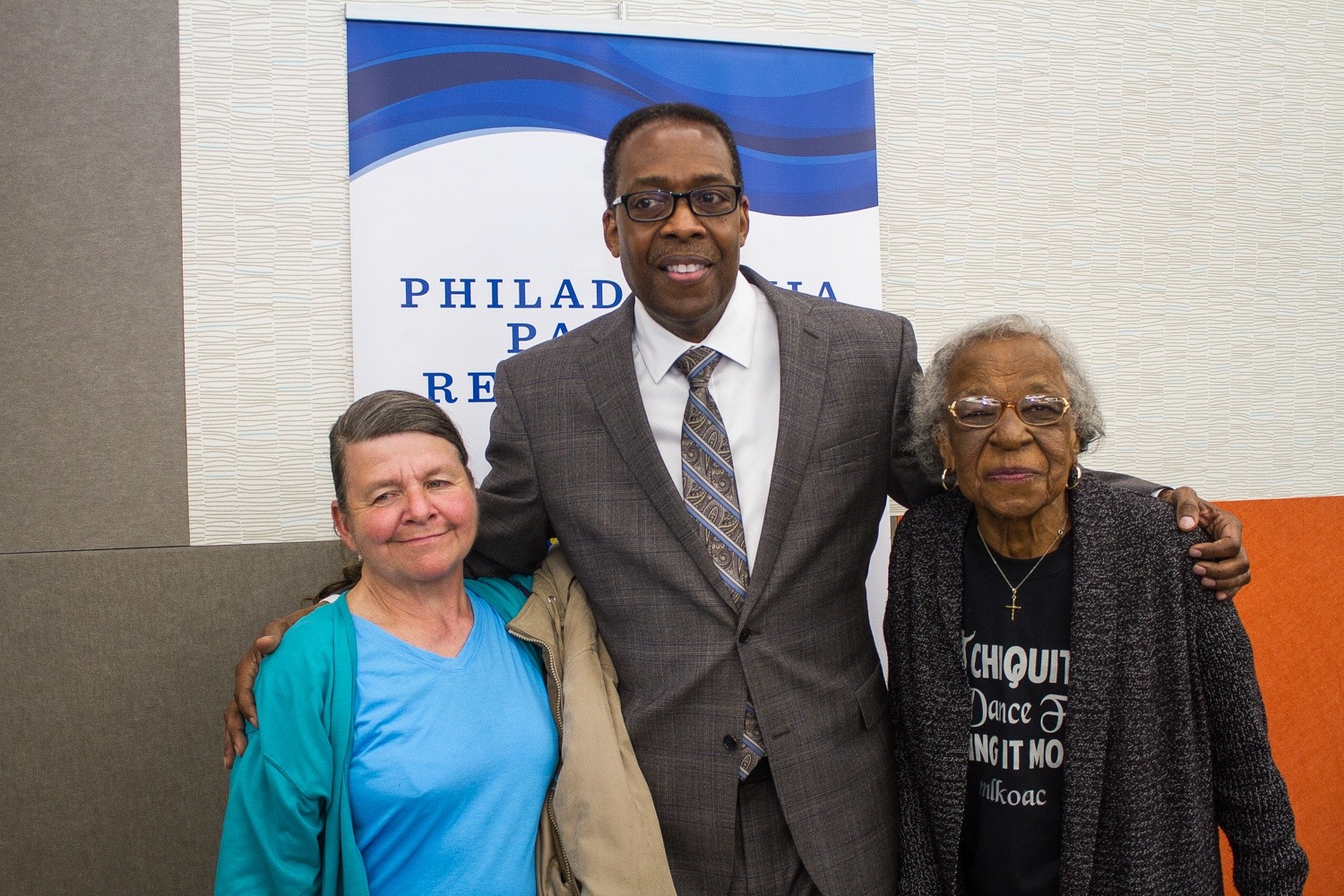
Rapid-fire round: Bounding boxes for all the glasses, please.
[611,185,741,222]
[941,392,1074,429]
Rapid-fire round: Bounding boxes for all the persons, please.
[881,315,1309,896]
[224,102,1252,896]
[213,391,676,896]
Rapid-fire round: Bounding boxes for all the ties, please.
[675,348,766,781]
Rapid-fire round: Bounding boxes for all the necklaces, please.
[977,494,1069,621]
